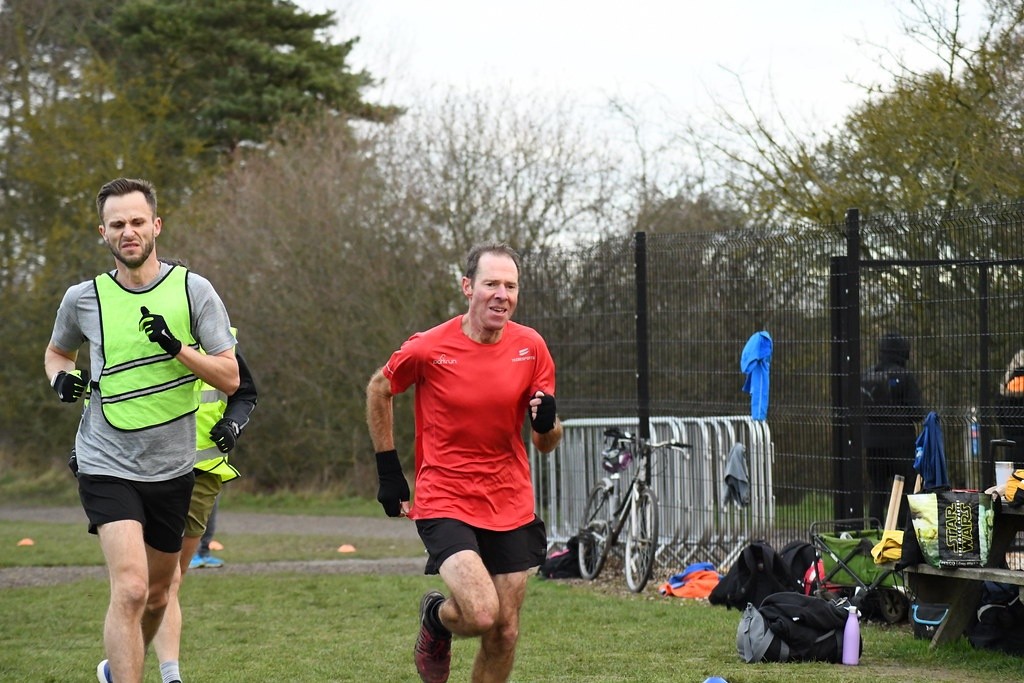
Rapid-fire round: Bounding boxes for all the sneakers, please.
[201,555,224,567]
[414,591,451,683]
[188,553,204,569]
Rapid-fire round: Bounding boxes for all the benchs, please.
[876,559,1024,649]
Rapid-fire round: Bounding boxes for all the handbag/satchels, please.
[907,485,995,568]
[736,596,863,664]
[912,602,950,640]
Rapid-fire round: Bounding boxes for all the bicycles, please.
[578,428,694,592]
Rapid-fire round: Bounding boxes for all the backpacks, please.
[803,557,850,598]
[708,539,781,611]
[779,539,816,579]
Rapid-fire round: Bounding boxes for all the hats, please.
[878,334,910,364]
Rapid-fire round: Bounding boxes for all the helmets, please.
[602,447,632,473]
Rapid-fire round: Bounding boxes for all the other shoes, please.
[96,659,113,683]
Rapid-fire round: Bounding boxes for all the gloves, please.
[138,306,182,358]
[50,369,89,403]
[210,417,241,454]
[68,446,78,478]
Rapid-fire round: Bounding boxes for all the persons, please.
[44,178,240,683]
[364,244,564,683]
[154,328,258,683]
[998,350,1024,463]
[861,334,927,530]
